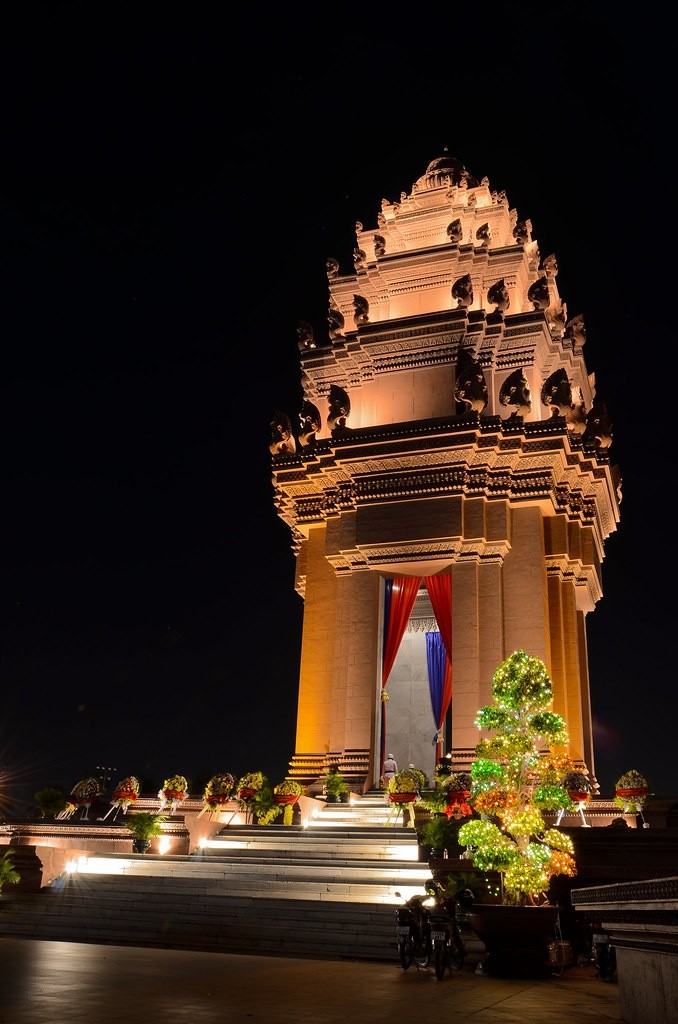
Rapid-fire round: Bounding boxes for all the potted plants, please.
[120,813,170,853]
[274,781,302,805]
[203,773,234,803]
[615,770,649,798]
[163,774,187,800]
[70,777,100,803]
[112,776,139,801]
[318,771,351,802]
[236,772,264,799]
[388,648,591,979]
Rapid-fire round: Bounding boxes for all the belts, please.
[385,771,394,773]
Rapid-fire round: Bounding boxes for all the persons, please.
[409,764,414,770]
[383,754,398,783]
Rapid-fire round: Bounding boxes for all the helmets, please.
[409,764,414,768]
[388,754,394,758]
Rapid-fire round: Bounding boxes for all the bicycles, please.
[390,890,467,982]
